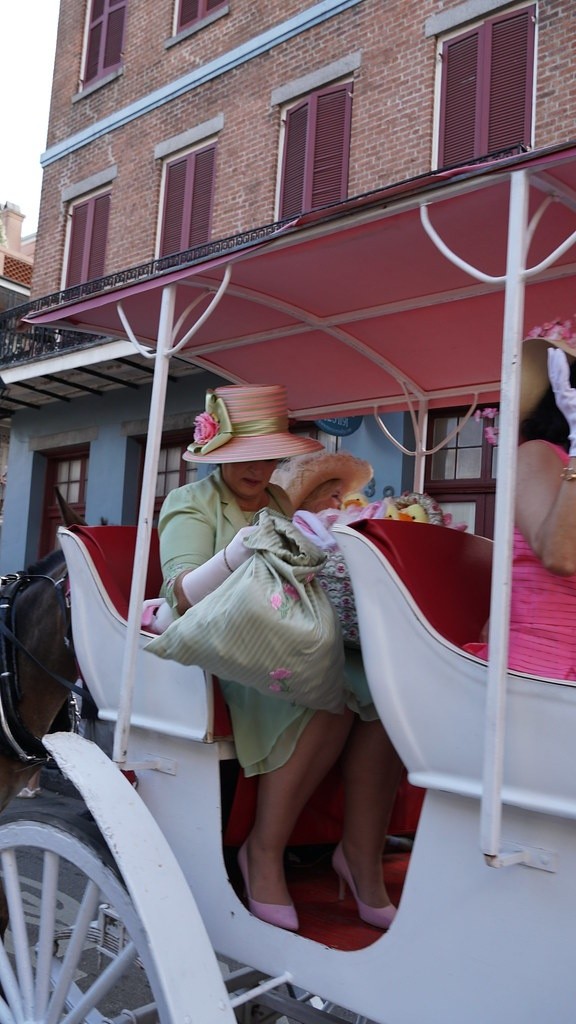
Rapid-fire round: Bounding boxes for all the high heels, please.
[332,841,397,930]
[236,839,299,931]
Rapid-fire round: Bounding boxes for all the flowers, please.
[476,408,500,446]
[193,414,219,444]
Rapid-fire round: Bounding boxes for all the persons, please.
[158,381,398,932]
[458,323,576,682]
[268,448,414,853]
[15,697,72,798]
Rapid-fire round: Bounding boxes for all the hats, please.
[181,383,326,464]
[519,316,576,425]
[270,447,374,512]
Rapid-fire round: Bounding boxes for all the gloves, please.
[546,348,576,457]
[182,525,258,606]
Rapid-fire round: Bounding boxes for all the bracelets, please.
[560,467,576,480]
[223,546,234,573]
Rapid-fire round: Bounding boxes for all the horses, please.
[0,470,110,814]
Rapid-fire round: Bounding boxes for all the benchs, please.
[326,519,576,823]
[58,522,236,743]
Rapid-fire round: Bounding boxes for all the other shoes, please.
[16,787,42,798]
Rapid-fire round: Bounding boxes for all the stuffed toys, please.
[340,490,427,521]
